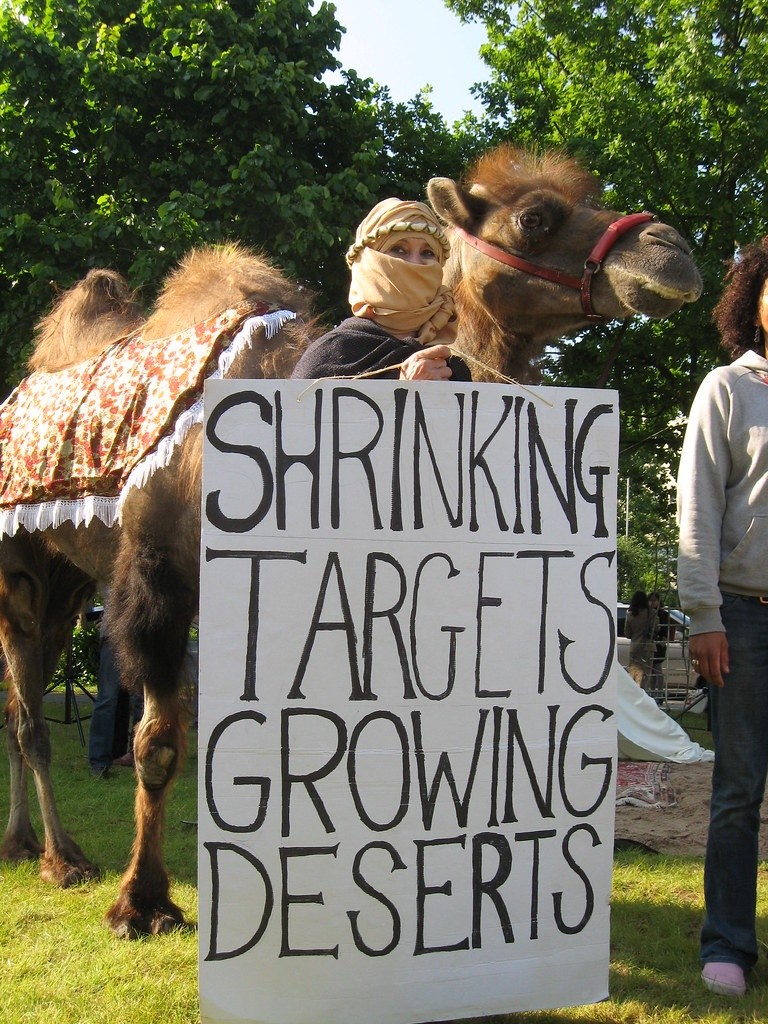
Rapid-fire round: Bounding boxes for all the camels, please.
[0,137,705,947]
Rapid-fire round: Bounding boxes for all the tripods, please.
[0,616,97,747]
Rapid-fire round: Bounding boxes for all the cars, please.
[617,602,710,700]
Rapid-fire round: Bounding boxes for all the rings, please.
[692,659,700,666]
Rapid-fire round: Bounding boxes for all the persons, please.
[290,196,473,382]
[623,232,768,996]
[82,618,149,778]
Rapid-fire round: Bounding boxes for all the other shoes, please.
[111,751,136,767]
[698,962,746,999]
